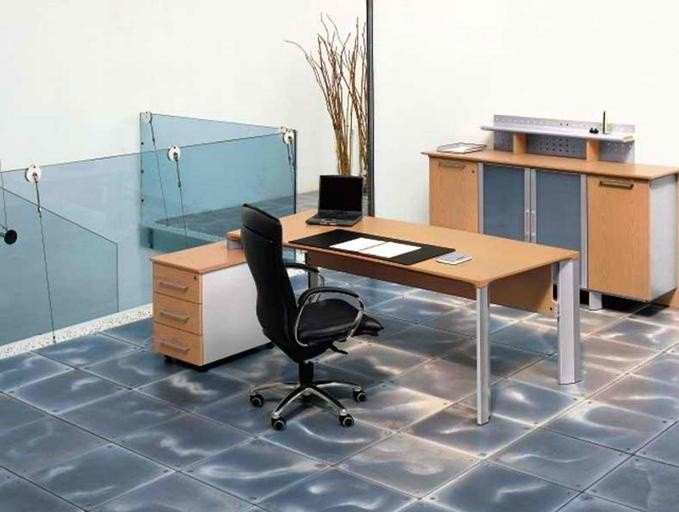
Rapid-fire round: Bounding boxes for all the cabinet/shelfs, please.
[421,145,678,310]
[149,237,274,368]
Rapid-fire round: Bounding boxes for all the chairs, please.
[239,203,383,431]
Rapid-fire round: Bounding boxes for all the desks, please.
[228,208,582,427]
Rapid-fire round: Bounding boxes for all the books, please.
[433,253,472,264]
[330,237,422,258]
